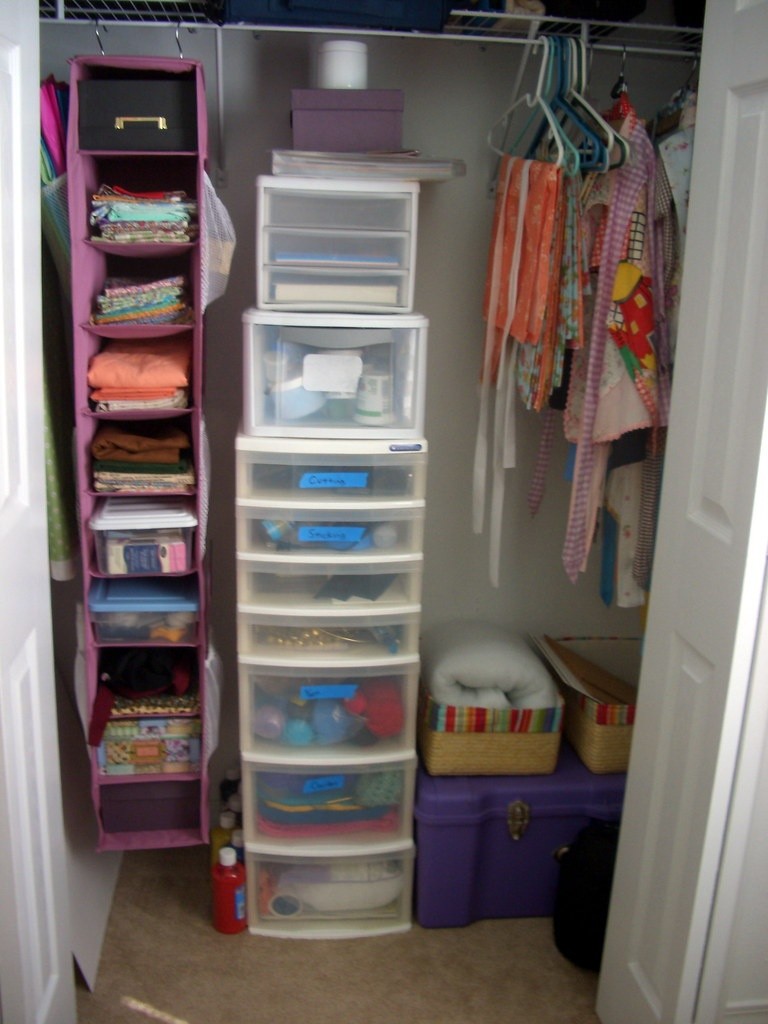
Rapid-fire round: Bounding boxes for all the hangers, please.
[484,32,703,174]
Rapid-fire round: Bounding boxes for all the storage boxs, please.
[239,308,429,440]
[86,497,198,574]
[411,734,627,928]
[87,576,199,643]
[77,76,197,150]
[290,87,403,151]
[99,780,199,834]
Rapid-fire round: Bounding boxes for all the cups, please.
[354,372,392,424]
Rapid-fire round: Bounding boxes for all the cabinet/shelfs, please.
[249,176,423,314]
[229,420,422,939]
[65,49,211,847]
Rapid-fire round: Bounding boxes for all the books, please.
[275,283,398,304]
[247,143,467,182]
[274,249,400,266]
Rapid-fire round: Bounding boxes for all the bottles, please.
[211,768,247,934]
